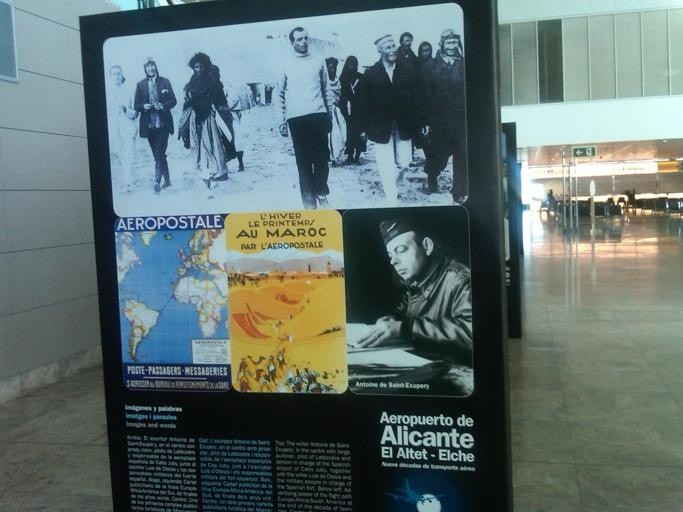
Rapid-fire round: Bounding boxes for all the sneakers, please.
[143,57,158,78]
[302,191,330,210]
[151,180,172,194]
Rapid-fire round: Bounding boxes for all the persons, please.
[109,52,244,199]
[357,212,471,369]
[265,27,467,208]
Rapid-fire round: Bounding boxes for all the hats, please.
[373,34,394,50]
[439,29,464,52]
[381,217,427,243]
